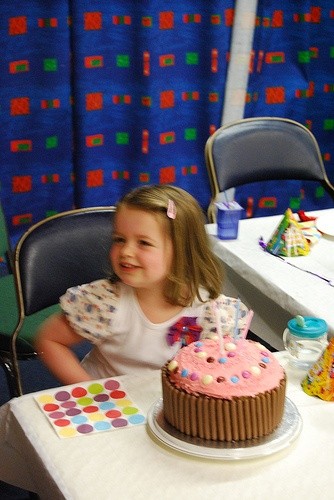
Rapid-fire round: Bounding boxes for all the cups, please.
[283,316,328,367]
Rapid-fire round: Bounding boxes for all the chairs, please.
[204,116,334,225]
[9,206,117,397]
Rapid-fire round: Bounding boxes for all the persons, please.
[33,184,251,385]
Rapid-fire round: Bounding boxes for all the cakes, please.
[161,335,287,441]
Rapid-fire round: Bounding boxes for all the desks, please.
[204,208,334,334]
[8,341,334,500]
